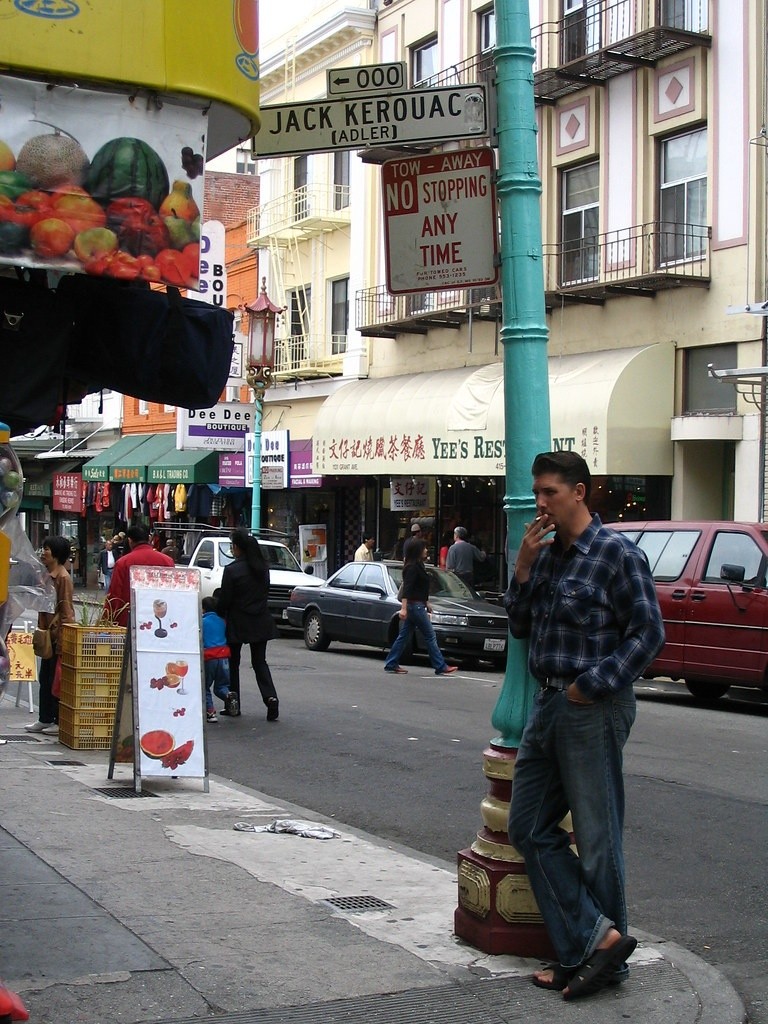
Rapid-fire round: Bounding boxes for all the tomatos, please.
[0,185,119,261]
[82,242,201,286]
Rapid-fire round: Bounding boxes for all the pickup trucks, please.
[174,535,327,627]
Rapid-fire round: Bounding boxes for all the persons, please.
[96,540,117,593]
[201,597,239,722]
[384,538,458,675]
[215,528,278,722]
[354,532,376,561]
[111,532,178,563]
[469,527,483,588]
[24,536,75,735]
[445,527,486,587]
[279,538,294,570]
[390,523,455,565]
[96,521,174,626]
[504,451,666,1002]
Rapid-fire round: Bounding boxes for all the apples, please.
[106,197,169,256]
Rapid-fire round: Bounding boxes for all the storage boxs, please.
[59,623,127,749]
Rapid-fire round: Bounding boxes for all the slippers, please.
[532,963,630,990]
[564,934,637,1000]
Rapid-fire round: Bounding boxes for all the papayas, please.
[159,180,200,229]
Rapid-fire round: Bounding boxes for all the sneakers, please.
[206,711,218,722]
[226,692,238,716]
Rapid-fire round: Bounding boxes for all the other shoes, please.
[220,709,241,716]
[26,721,53,733]
[267,698,278,720]
[440,665,458,673]
[41,724,59,735]
[395,668,409,673]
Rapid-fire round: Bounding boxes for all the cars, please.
[285,560,509,668]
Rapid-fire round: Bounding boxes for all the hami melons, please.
[0,139,17,172]
[15,129,92,187]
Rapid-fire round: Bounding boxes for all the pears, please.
[163,208,201,248]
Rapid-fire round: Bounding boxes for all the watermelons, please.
[85,136,169,210]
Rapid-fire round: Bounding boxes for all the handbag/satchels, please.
[52,654,60,699]
[32,627,53,659]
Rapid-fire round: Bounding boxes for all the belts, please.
[108,568,113,569]
[541,686,559,692]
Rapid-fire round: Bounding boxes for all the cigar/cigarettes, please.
[535,516,543,520]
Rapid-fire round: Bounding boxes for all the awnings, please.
[24,462,81,496]
[311,339,677,476]
[33,427,105,458]
[82,433,219,483]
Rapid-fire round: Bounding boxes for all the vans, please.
[602,520,768,701]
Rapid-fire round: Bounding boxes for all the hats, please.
[411,523,424,532]
